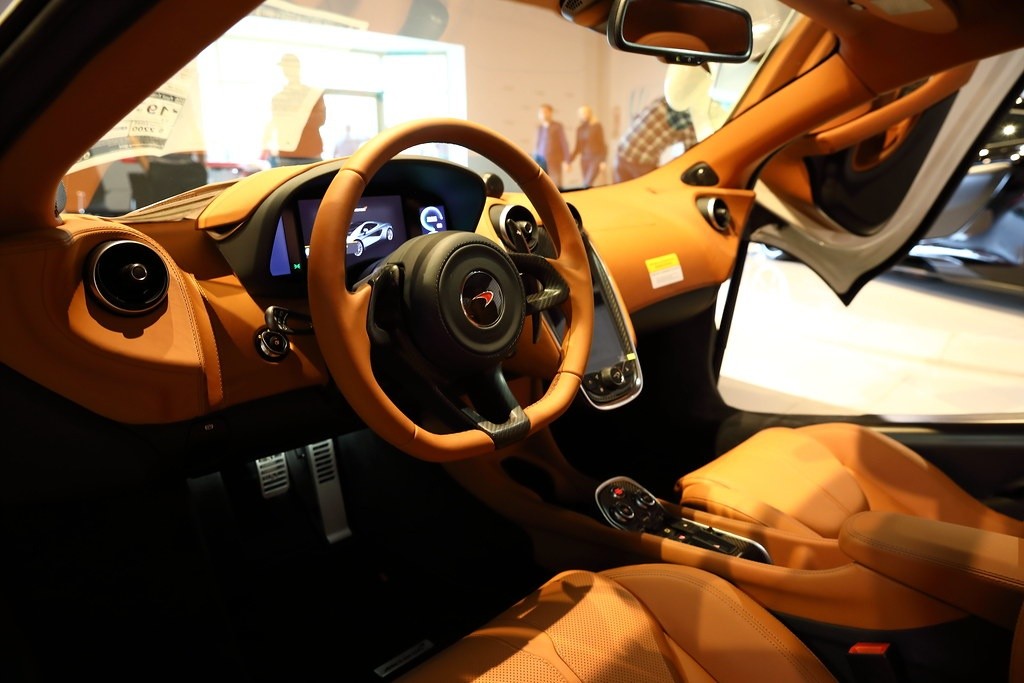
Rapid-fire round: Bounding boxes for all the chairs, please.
[409,560,846,682]
[657,422,1024,628]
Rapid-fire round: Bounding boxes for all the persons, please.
[564,104,608,189]
[535,104,572,190]
[258,54,328,168]
[613,94,699,184]
